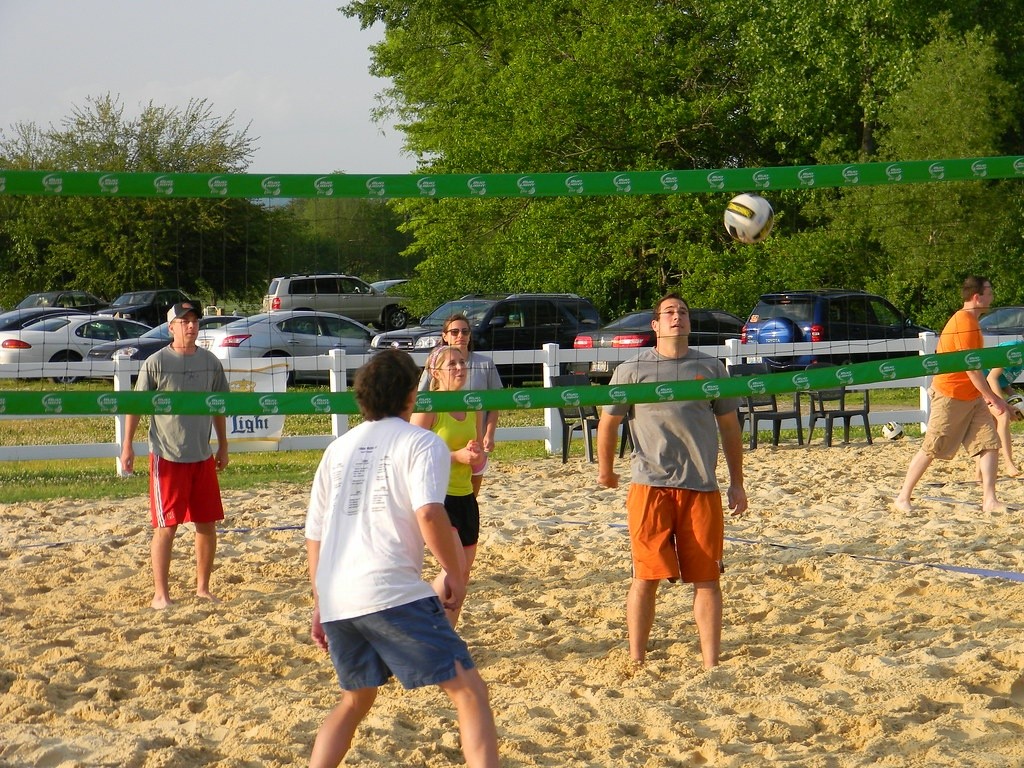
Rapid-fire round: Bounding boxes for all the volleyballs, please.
[1006,394,1024,422]
[723,193,775,243]
[881,421,905,441]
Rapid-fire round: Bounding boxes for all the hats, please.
[166,302,196,326]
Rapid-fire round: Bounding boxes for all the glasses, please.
[445,328,471,337]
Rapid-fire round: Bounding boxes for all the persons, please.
[120,303,230,610]
[418,314,503,498]
[597,293,747,672]
[232,309,238,316]
[893,275,1007,514]
[411,346,484,629]
[975,338,1024,486]
[305,349,499,768]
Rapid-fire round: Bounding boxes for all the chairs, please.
[728,361,804,450]
[550,374,635,462]
[805,363,873,447]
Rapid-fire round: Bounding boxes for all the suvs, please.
[262,275,410,330]
[743,290,936,373]
[368,293,605,387]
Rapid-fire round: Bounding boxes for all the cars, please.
[570,309,746,385]
[0,307,91,331]
[194,311,381,386]
[0,314,171,384]
[95,290,202,327]
[85,316,243,381]
[20,292,114,312]
[978,306,1024,386]
